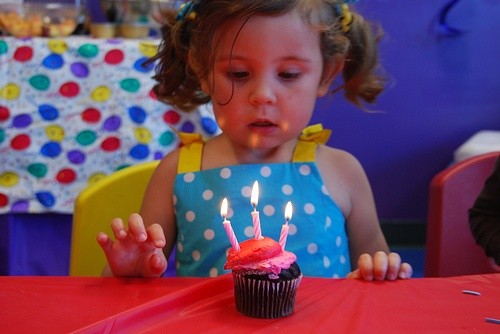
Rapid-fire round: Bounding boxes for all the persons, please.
[97,0,413,281]
[468,156,500,266]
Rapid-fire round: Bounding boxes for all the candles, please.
[219,197,240,251]
[279,201,293,251]
[251,180,262,239]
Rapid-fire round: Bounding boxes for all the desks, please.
[0,274,499,334]
[0,35,223,276]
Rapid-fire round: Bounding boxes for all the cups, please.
[0,4,151,40]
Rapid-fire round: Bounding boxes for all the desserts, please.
[225,236,303,318]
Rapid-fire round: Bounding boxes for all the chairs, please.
[67,158,162,277]
[427,151,500,278]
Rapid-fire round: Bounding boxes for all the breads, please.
[0,10,76,38]
